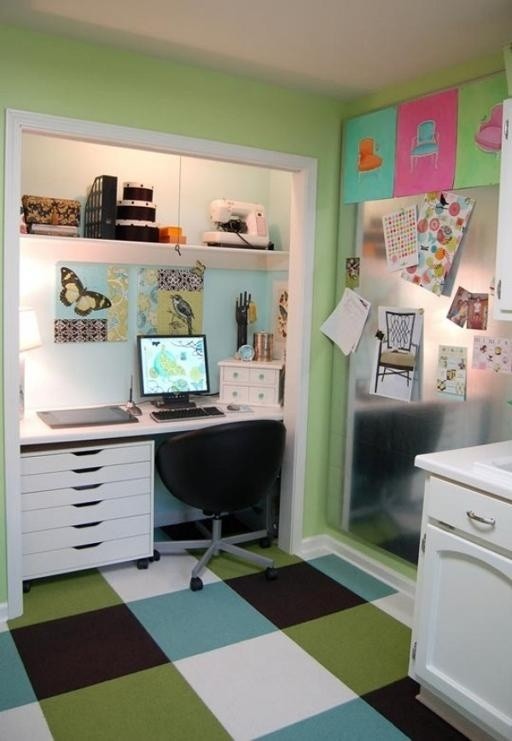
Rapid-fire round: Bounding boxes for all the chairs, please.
[152,420,285,592]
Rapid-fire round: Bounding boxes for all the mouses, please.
[227,403,240,410]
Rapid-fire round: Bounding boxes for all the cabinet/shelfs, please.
[407,440,512,741]
[493,99,512,322]
[4,109,319,623]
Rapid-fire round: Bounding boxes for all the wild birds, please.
[169,293,196,335]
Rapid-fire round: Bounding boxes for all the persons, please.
[233,290,253,353]
[448,296,485,330]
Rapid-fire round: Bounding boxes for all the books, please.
[28,224,82,238]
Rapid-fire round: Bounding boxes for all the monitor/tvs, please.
[137,334,210,409]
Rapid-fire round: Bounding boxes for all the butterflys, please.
[58,267,112,316]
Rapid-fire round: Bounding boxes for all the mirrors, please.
[346,182,512,571]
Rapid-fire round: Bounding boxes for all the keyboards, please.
[150,407,226,423]
